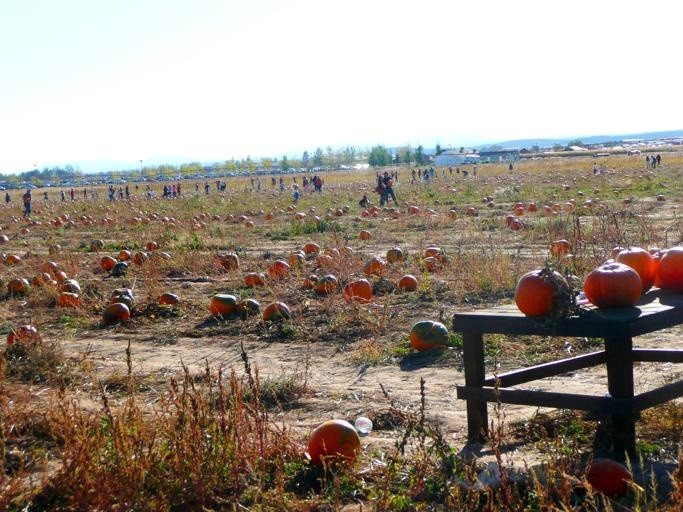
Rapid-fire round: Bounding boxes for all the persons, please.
[593,162,597,175]
[359,195,369,210]
[646,154,661,169]
[91,187,95,198]
[61,190,66,202]
[70,188,74,201]
[44,191,48,200]
[372,170,400,208]
[251,174,322,204]
[5,192,10,203]
[411,164,477,180]
[600,164,604,173]
[83,188,87,198]
[23,190,31,218]
[108,181,226,204]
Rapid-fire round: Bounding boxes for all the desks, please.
[445,283,683,454]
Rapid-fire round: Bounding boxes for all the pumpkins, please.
[551,239,571,256]
[585,459,632,497]
[309,419,361,469]
[606,247,682,293]
[515,268,570,318]
[657,195,666,202]
[1,179,477,320]
[411,321,449,352]
[584,263,641,309]
[7,326,43,346]
[479,179,632,230]
